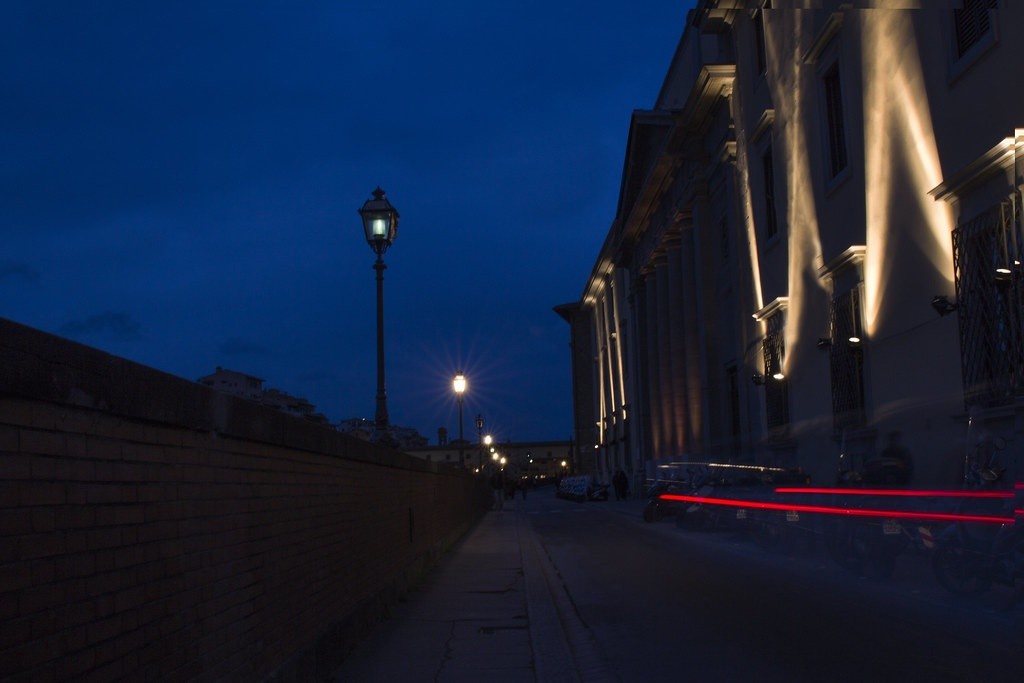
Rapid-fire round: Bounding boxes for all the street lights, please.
[450,370,470,471]
[475,414,485,472]
[358,186,401,443]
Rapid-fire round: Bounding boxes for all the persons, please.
[492,473,564,501]
[612,470,629,502]
[874,434,915,489]
